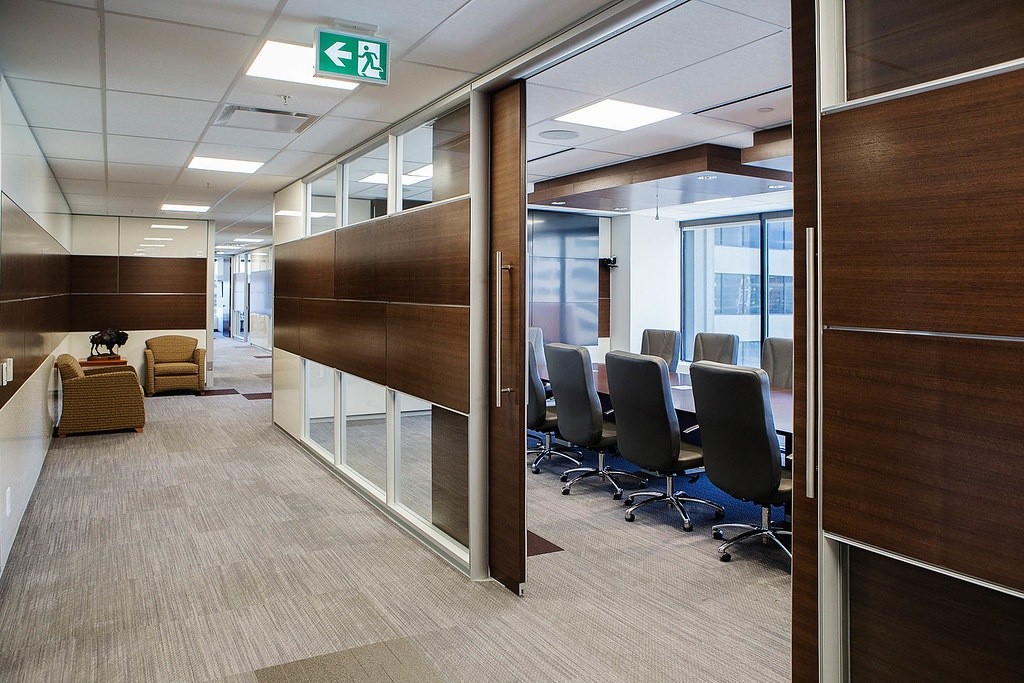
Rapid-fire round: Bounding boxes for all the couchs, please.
[54,354,145,437]
[145,335,209,396]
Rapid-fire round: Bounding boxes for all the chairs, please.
[527,326,793,576]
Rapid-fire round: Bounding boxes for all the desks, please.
[536,362,793,480]
[76,357,129,366]
